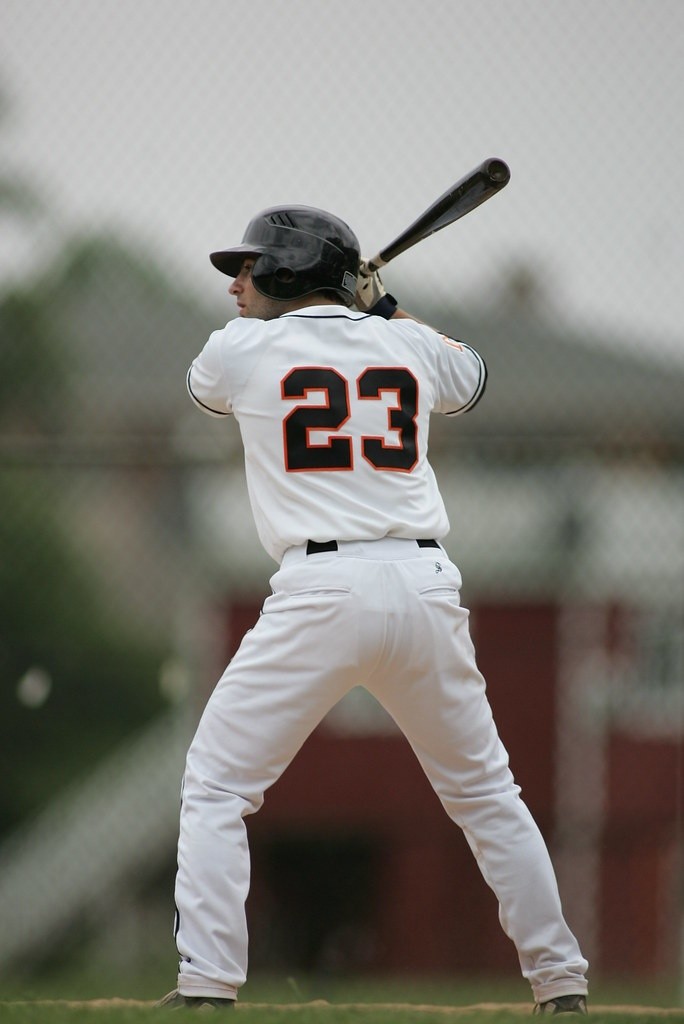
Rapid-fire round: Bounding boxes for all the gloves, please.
[357,258,398,324]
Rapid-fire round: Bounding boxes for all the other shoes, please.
[532,995,589,1019]
[152,989,235,1014]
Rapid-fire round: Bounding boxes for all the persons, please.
[150,209,590,1016]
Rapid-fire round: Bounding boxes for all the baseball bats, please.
[355,155,512,275]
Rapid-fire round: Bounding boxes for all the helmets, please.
[209,204,363,307]
